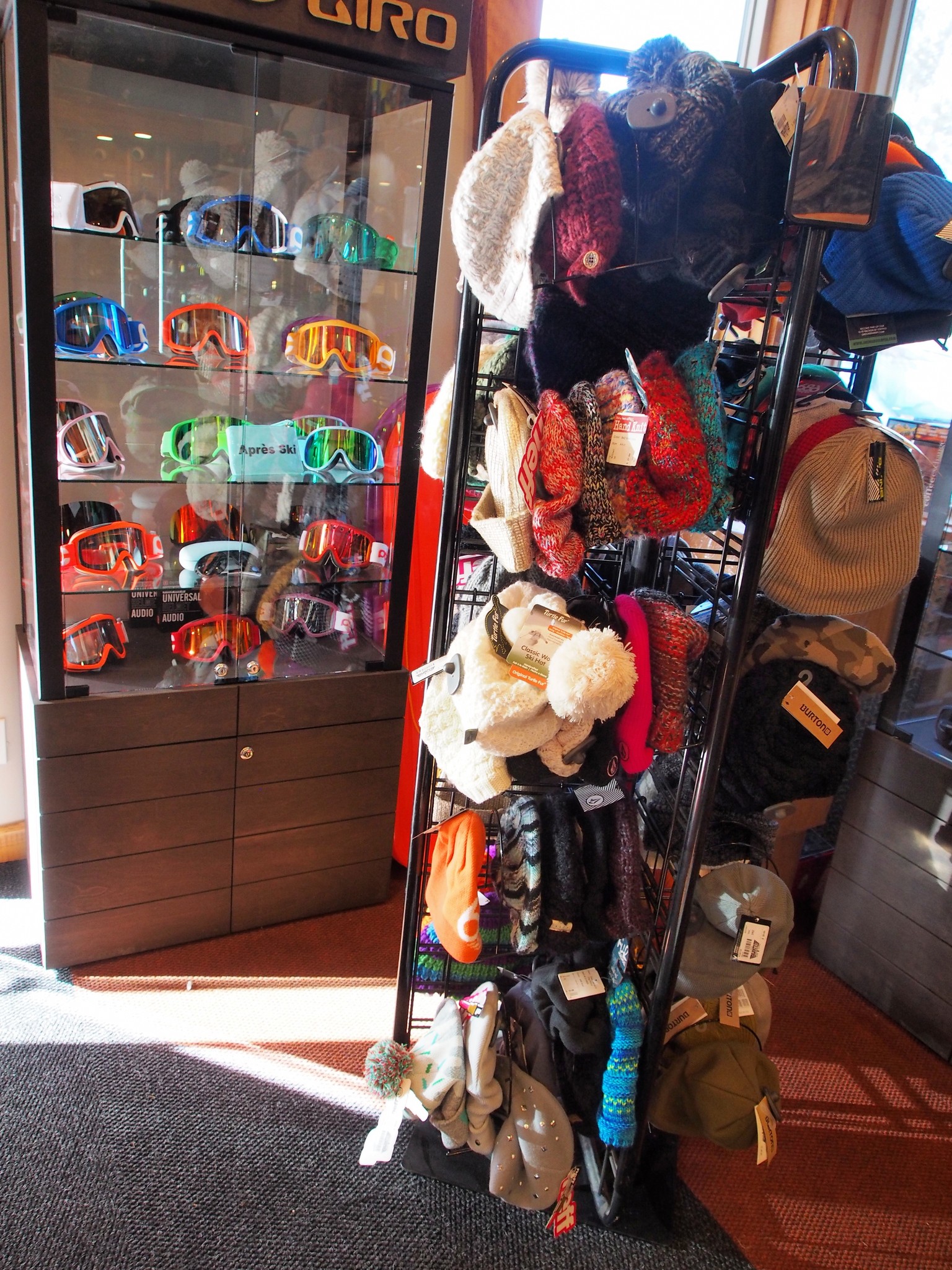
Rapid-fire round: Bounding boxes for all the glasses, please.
[293,415,350,438]
[188,195,302,257]
[299,520,375,568]
[161,458,232,482]
[63,614,129,670]
[270,593,339,637]
[283,316,379,375]
[179,541,263,576]
[160,416,255,465]
[164,305,255,359]
[170,500,248,544]
[82,181,140,238]
[55,292,134,358]
[303,470,376,485]
[303,426,378,475]
[60,521,163,574]
[302,214,399,269]
[57,398,125,467]
[170,614,261,663]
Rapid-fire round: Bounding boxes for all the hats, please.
[369,798,596,1211]
[416,36,791,808]
[642,111,952,1149]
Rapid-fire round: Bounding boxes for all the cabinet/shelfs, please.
[806,421,952,1072]
[0,0,469,970]
